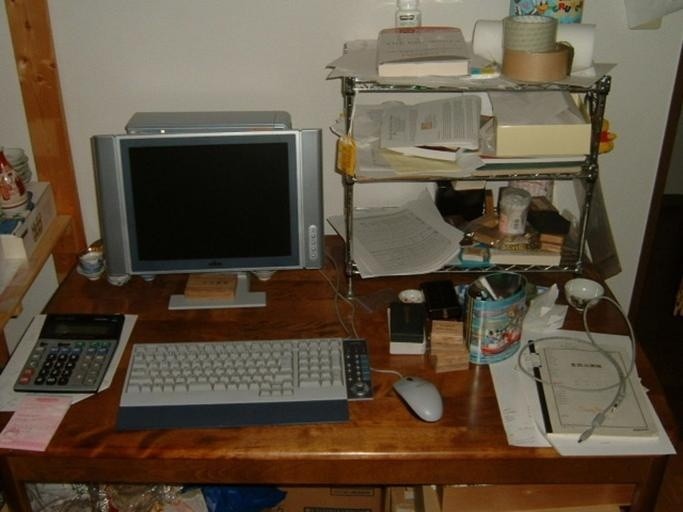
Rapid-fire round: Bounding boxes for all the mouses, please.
[391,373,444,423]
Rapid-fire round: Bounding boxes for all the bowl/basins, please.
[76,265,106,281]
[564,277,605,311]
[251,271,276,281]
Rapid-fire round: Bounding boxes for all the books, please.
[528,340,660,439]
[375,26,473,79]
[488,248,562,267]
[386,115,494,163]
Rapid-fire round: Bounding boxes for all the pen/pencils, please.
[468,276,504,301]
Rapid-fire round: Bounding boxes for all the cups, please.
[78,252,104,273]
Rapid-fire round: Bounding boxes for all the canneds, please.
[463,271,528,365]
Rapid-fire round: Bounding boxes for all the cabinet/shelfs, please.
[343,41,612,299]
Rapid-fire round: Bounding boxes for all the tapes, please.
[503,14,570,84]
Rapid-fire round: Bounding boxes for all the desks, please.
[0,234,681,512]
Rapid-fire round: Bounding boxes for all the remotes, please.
[342,338,376,401]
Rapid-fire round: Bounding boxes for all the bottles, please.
[498,187,532,236]
[395,0,422,28]
[0,147,30,216]
[4,148,32,183]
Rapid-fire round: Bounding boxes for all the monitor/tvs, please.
[90,111,324,310]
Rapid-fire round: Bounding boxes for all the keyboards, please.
[119,337,348,408]
